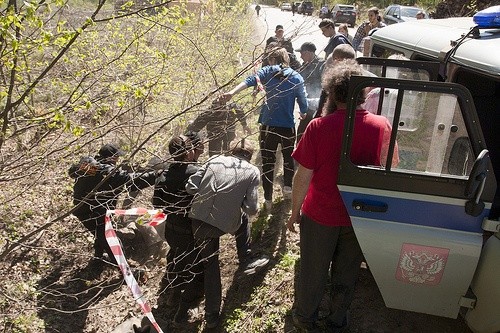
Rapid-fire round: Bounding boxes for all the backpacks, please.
[69,154,116,180]
[152,164,198,206]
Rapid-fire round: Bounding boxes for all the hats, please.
[298,44,316,52]
[100,143,126,157]
[276,25,283,31]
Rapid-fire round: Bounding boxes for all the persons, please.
[280,58,399,333]
[222,47,308,210]
[333,44,404,114]
[186,99,251,157]
[292,2,295,15]
[302,1,307,14]
[261,25,326,144]
[255,5,261,15]
[153,133,212,308]
[354,7,386,52]
[417,11,425,20]
[319,18,353,57]
[74,144,162,263]
[184,138,261,315]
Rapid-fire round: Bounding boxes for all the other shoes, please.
[264,199,272,210]
[239,240,262,263]
[291,309,348,333]
[282,185,292,200]
[205,310,219,328]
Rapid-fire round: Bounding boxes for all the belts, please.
[164,221,192,234]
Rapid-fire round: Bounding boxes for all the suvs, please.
[330,5,357,28]
[297,1,314,16]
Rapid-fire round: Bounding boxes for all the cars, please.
[384,6,429,24]
[293,2,302,11]
[319,8,331,19]
[280,3,292,11]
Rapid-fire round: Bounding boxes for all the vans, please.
[315,5,500,325]
